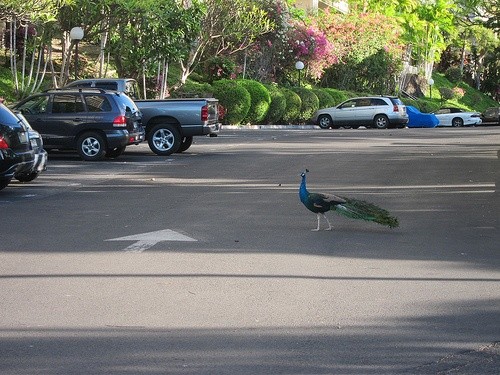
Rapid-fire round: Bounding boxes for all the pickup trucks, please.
[64,79,220,155]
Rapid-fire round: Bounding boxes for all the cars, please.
[431,106,482,127]
[0,104,35,191]
[407,106,440,127]
[312,95,409,129]
[10,109,48,182]
[480,106,500,125]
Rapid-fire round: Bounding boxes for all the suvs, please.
[12,88,145,161]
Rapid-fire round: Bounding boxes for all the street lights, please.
[70,27,85,80]
[295,61,305,88]
[427,78,435,98]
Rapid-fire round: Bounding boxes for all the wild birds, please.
[296,169,401,231]
